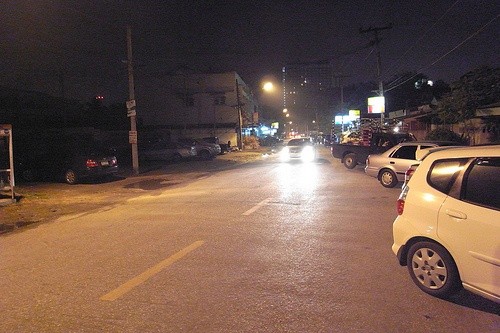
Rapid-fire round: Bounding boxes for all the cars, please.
[182,135,222,161]
[287,137,311,159]
[13,138,118,184]
[365,142,441,188]
[402,147,458,190]
[138,136,197,161]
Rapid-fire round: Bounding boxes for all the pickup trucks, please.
[331,131,418,170]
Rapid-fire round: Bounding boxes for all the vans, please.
[392,146,500,306]
